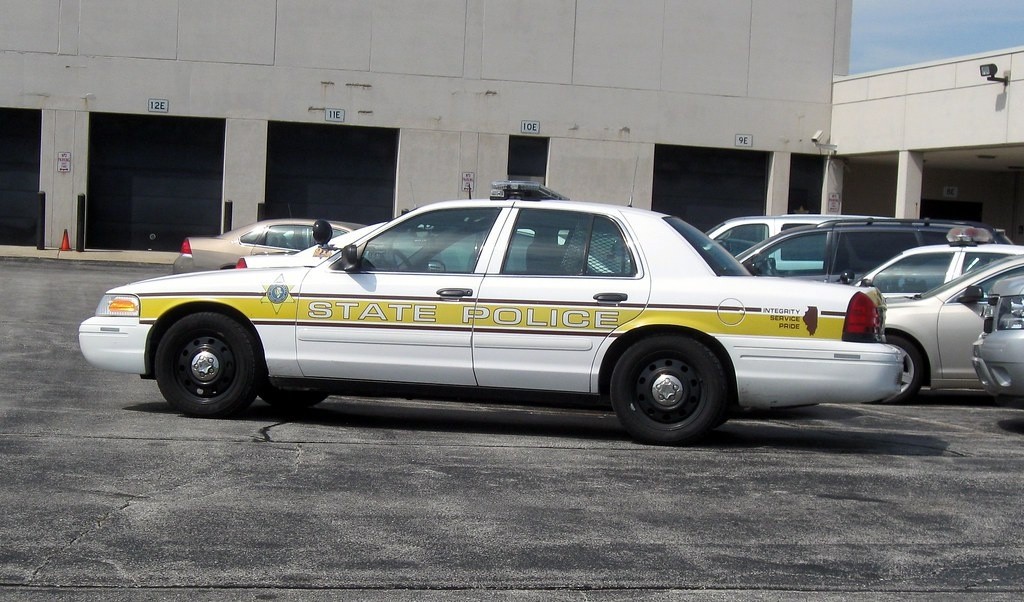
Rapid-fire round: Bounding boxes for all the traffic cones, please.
[59,229,72,251]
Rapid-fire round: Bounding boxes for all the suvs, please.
[705,214,1024,405]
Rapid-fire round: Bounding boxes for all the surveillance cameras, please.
[811,129,823,143]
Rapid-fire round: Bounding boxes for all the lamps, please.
[980,64,1009,86]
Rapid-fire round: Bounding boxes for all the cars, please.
[77,180,904,446]
[173,218,368,274]
[236,222,617,275]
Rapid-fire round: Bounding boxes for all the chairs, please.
[520,241,561,276]
